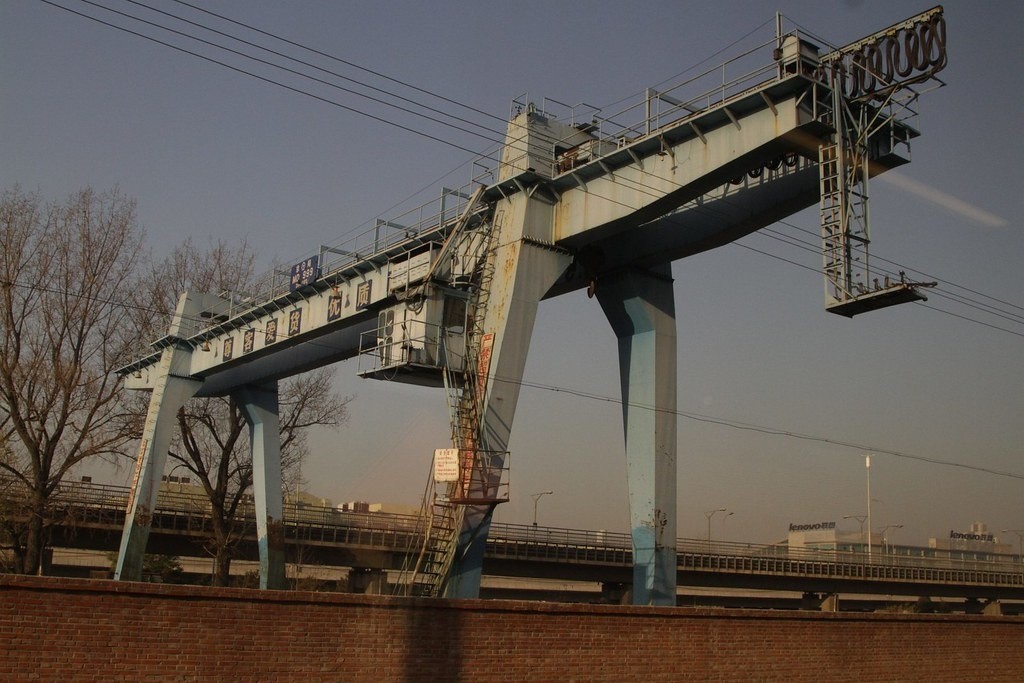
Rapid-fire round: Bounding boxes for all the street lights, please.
[703,509,723,567]
[531,490,553,536]
[1003,529,1023,569]
[718,512,735,567]
[845,516,868,561]
[861,454,878,562]
[880,525,903,564]
